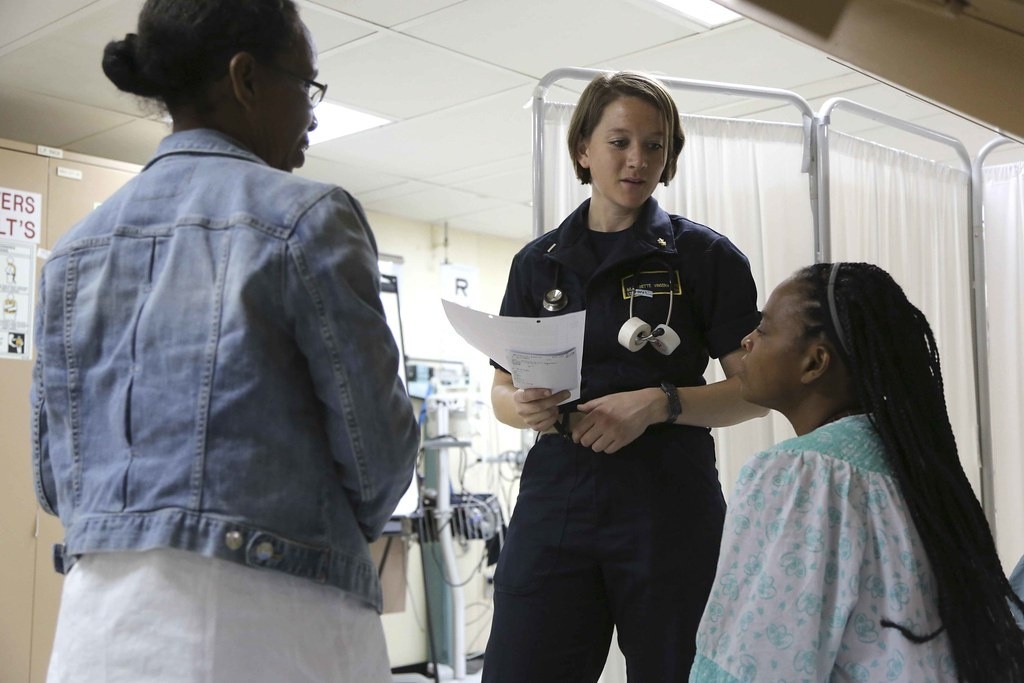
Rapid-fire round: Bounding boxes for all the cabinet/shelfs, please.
[0,137,152,683]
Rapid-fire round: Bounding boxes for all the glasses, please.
[279,70,327,107]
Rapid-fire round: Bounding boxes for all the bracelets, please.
[660,383,682,423]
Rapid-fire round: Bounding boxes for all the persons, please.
[688,262,1024,683]
[482,71,770,683]
[9,335,24,354]
[31,0,420,683]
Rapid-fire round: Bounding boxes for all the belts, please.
[539,409,593,437]
